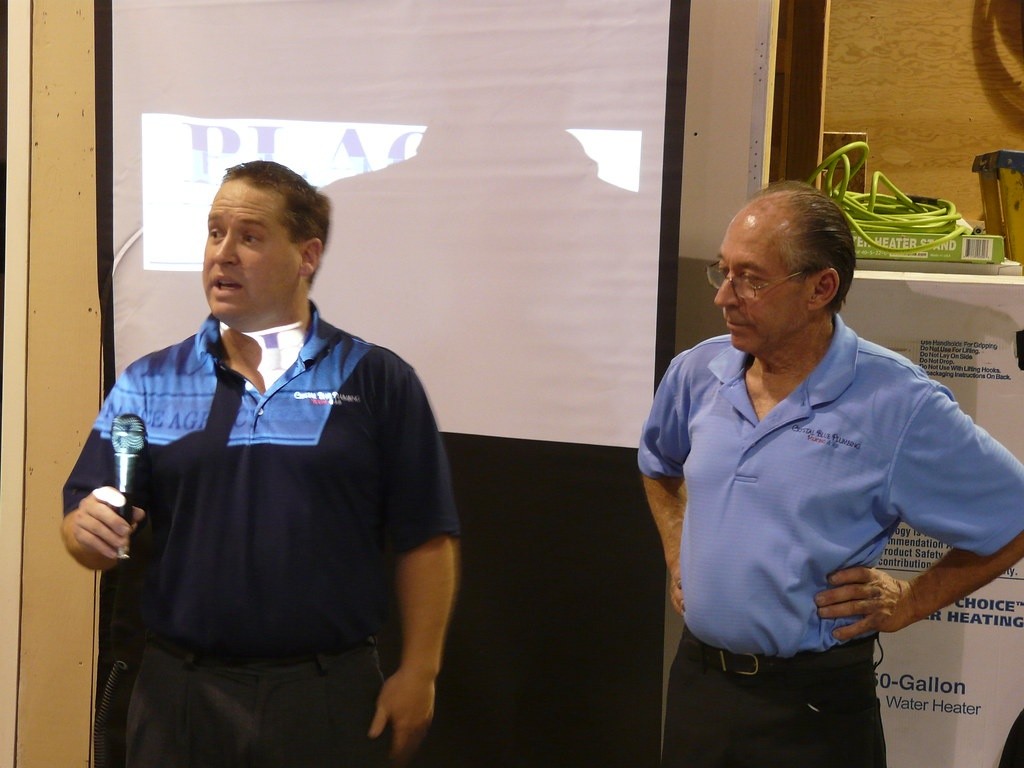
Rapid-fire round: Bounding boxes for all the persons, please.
[637,184,1024,768]
[61,160,459,768]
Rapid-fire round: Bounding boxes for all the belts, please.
[681,622,875,677]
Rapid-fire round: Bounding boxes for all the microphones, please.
[110,413,148,562]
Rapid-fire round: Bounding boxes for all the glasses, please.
[706,259,815,300]
